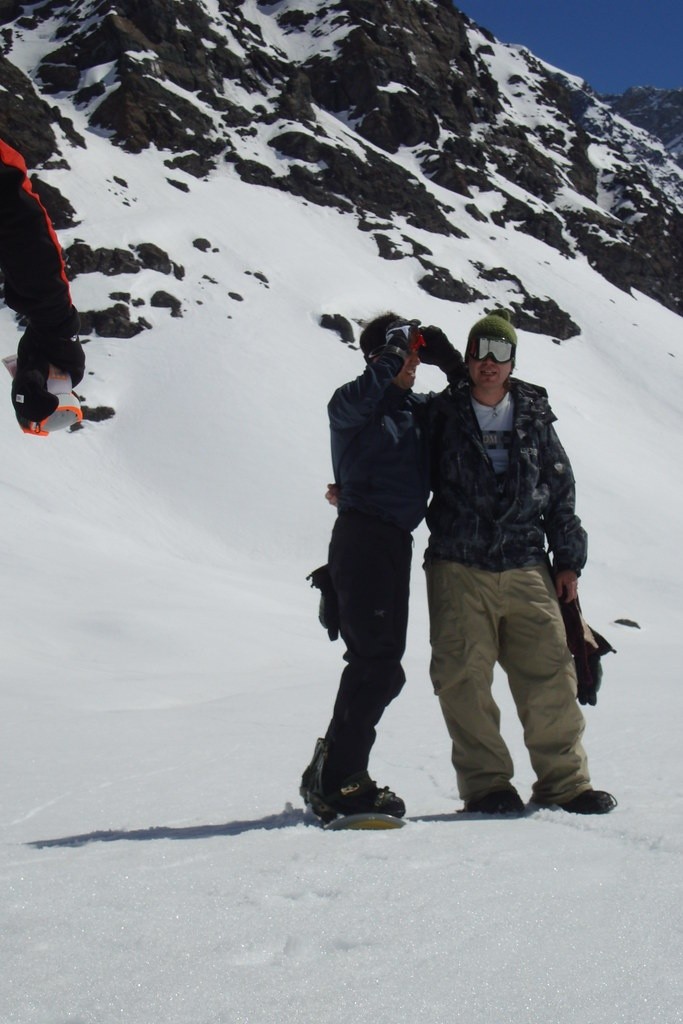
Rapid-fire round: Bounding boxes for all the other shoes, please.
[299,777,406,823]
[466,792,525,813]
[564,788,618,815]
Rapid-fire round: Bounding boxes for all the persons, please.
[0,140,86,437]
[325,308,618,817]
[299,310,465,817]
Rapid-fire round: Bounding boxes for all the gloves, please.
[384,317,422,364]
[415,325,460,371]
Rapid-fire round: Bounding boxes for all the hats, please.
[465,308,517,365]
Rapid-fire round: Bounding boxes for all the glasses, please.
[467,336,518,363]
